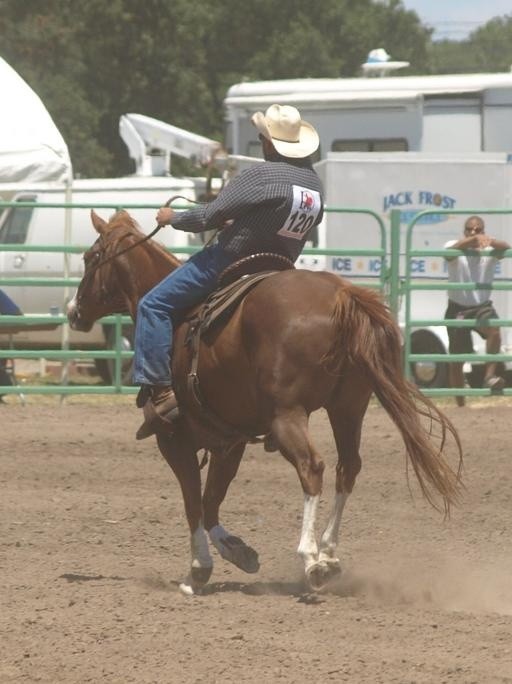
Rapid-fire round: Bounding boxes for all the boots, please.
[135,383,181,440]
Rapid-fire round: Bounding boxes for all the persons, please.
[130,104,325,440]
[0,290,23,316]
[441,215,511,407]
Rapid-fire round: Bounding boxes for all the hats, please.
[250,104,320,158]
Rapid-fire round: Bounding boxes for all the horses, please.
[64,205,468,599]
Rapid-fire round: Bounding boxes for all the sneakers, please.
[483,376,506,389]
